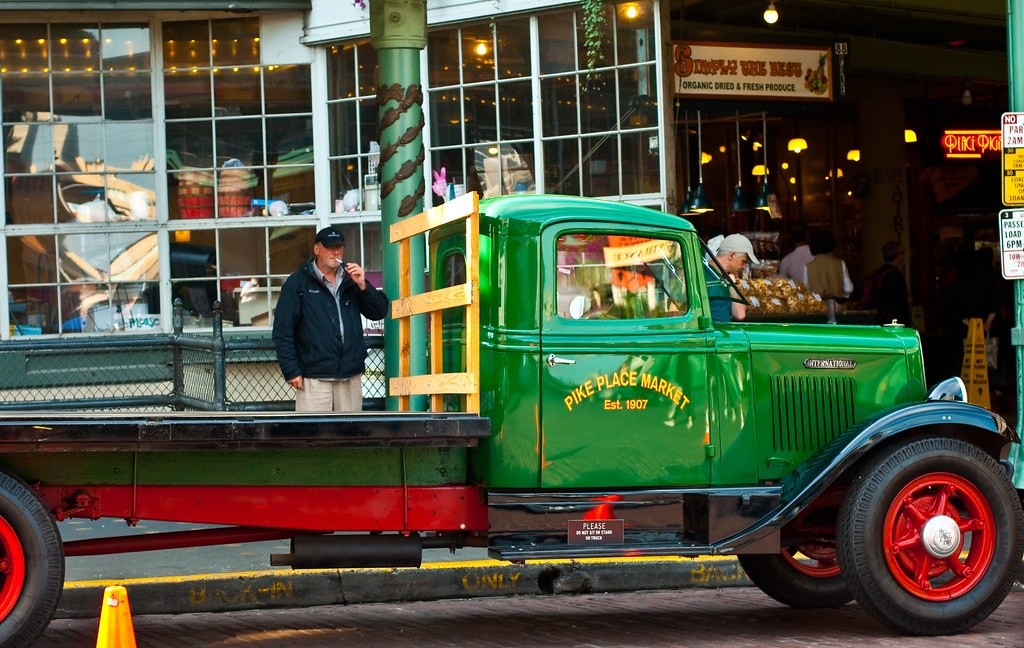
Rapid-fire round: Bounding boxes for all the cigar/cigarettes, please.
[336,259,343,263]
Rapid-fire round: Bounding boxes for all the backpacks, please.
[855,266,896,317]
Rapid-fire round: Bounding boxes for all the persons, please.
[778,220,816,286]
[928,242,1014,397]
[271,227,390,412]
[668,232,760,322]
[802,229,854,302]
[855,240,916,329]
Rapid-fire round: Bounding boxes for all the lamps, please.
[701,152,711,164]
[752,165,770,175]
[905,130,916,142]
[788,138,807,153]
[962,90,971,105]
[754,111,773,211]
[630,114,648,125]
[475,33,489,55]
[625,2,639,18]
[730,111,754,211]
[847,150,860,161]
[677,111,701,216]
[829,168,843,178]
[764,2,778,23]
[688,110,715,213]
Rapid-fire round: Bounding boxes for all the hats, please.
[316,227,346,248]
[719,233,760,264]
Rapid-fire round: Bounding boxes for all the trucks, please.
[0,189,1023,647]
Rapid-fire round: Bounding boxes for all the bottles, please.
[739,231,783,260]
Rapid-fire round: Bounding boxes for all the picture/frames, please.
[633,27,650,98]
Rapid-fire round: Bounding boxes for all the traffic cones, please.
[95,585,140,647]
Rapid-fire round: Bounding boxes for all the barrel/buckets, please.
[178,166,253,219]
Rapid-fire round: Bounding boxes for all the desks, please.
[737,310,879,326]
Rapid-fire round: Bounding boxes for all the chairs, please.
[146,242,284,324]
